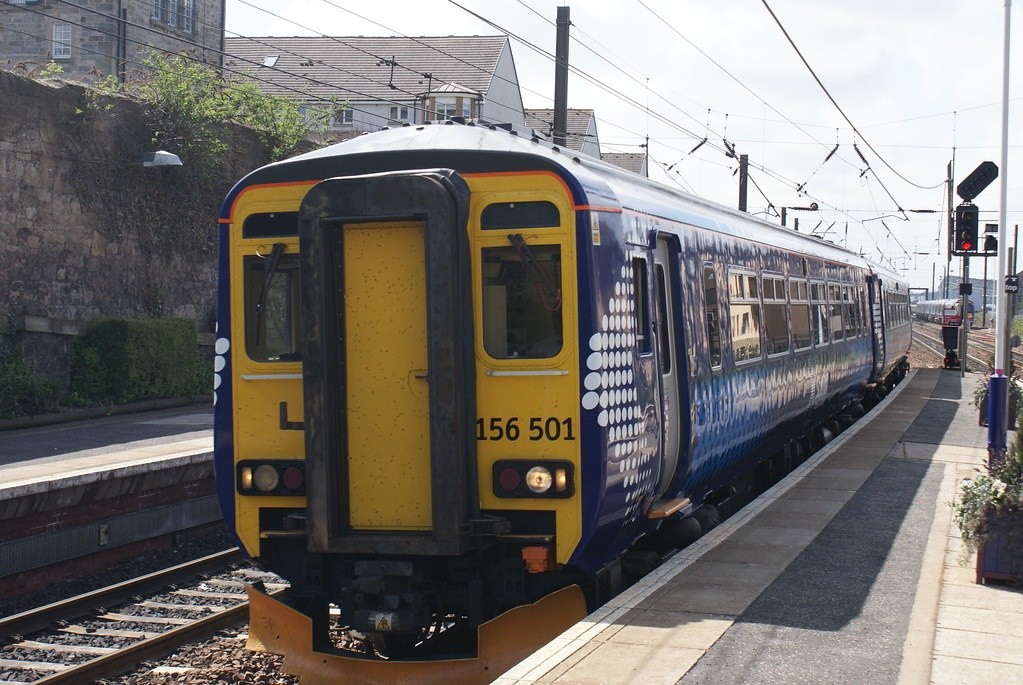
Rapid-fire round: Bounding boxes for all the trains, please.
[915,298,975,330]
[209,116,914,684]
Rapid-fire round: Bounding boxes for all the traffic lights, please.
[959,162,998,200]
[958,283,972,294]
[954,204,977,253]
